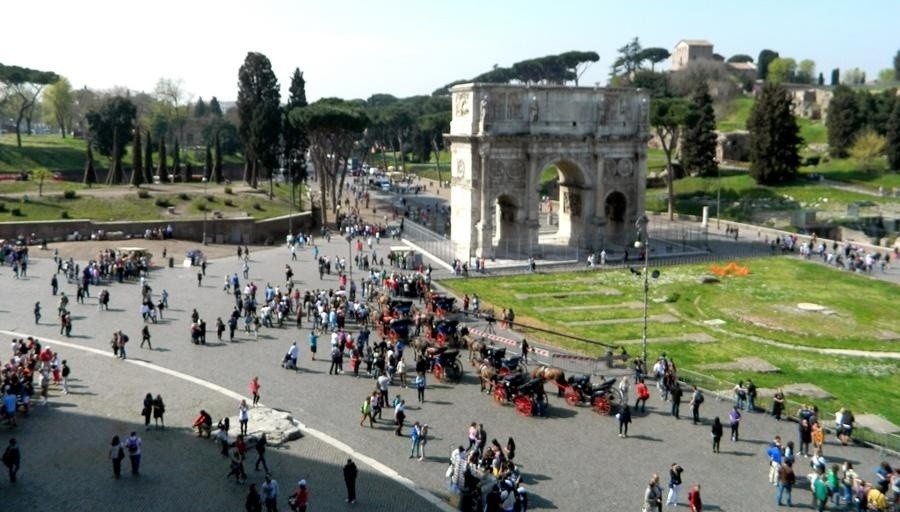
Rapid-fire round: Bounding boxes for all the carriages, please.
[367,291,620,420]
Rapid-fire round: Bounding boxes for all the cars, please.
[325,151,391,192]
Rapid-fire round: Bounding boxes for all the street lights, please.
[286,150,299,245]
[714,133,727,231]
[197,144,209,247]
[636,214,655,372]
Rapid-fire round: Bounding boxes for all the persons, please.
[771,232,890,273]
[588,248,644,266]
[547,200,553,211]
[527,256,535,271]
[1,223,246,511]
[225,256,528,511]
[600,347,900,510]
[286,170,450,279]
